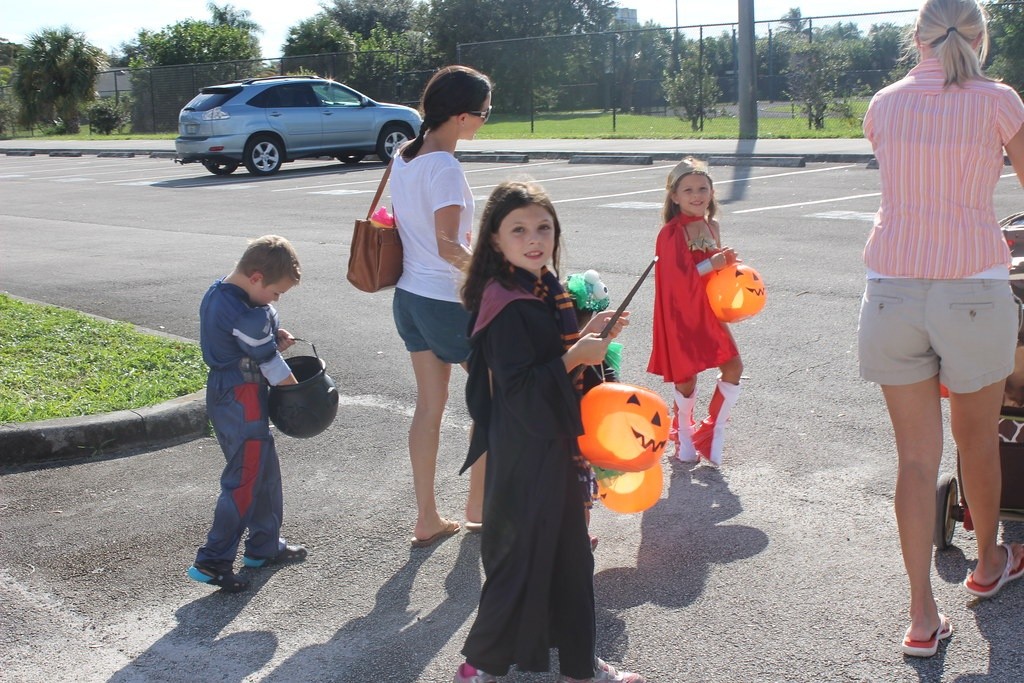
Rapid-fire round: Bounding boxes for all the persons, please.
[456,173,646,681]
[561,283,618,552]
[645,155,746,465]
[187,234,310,594]
[852,0,1024,661]
[382,63,491,548]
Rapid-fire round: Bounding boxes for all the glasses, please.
[457,105,493,123]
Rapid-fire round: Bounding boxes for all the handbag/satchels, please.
[346,141,404,292]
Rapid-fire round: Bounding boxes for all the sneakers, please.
[558,655,644,683]
[453,663,497,683]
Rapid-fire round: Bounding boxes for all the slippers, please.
[902,543,1024,656]
[410,521,488,547]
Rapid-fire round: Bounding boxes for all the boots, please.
[667,372,742,465]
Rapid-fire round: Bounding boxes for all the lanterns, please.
[576,376,670,471]
[592,458,665,514]
[707,262,766,323]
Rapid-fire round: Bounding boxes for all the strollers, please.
[932,213,1024,551]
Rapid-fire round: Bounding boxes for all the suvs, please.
[173,74,425,177]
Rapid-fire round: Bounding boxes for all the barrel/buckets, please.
[269,335,340,440]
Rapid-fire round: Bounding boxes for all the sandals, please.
[187,561,250,592]
[244,545,307,567]
